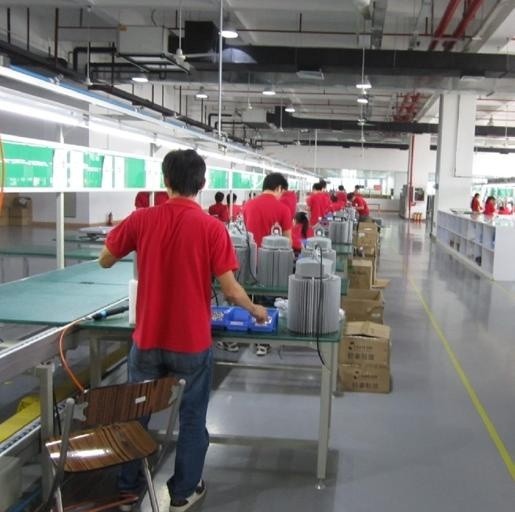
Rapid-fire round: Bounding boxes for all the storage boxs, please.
[339,320,390,395]
[356,221,379,257]
[347,259,373,290]
[353,255,391,287]
[341,288,386,324]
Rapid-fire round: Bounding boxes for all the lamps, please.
[263,78,275,95]
[132,69,148,83]
[196,86,208,99]
[355,62,373,89]
[218,4,239,39]
[357,92,369,104]
[284,101,295,114]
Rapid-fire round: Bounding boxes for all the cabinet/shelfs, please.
[436,209,514,283]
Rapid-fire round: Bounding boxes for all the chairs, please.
[41,375,186,512]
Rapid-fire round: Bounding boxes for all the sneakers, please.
[169,478,207,512]
[254,343,272,357]
[117,477,150,512]
[215,341,239,353]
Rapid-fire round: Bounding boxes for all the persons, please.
[472,193,515,215]
[306,180,369,231]
[98,149,268,512]
[207,193,227,221]
[243,173,292,248]
[289,213,312,252]
[135,192,169,209]
[226,194,240,223]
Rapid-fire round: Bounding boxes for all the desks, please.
[80,299,346,490]
[331,259,346,273]
[325,242,354,254]
[208,277,349,359]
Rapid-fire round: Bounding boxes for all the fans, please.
[65,8,122,88]
[148,8,217,70]
